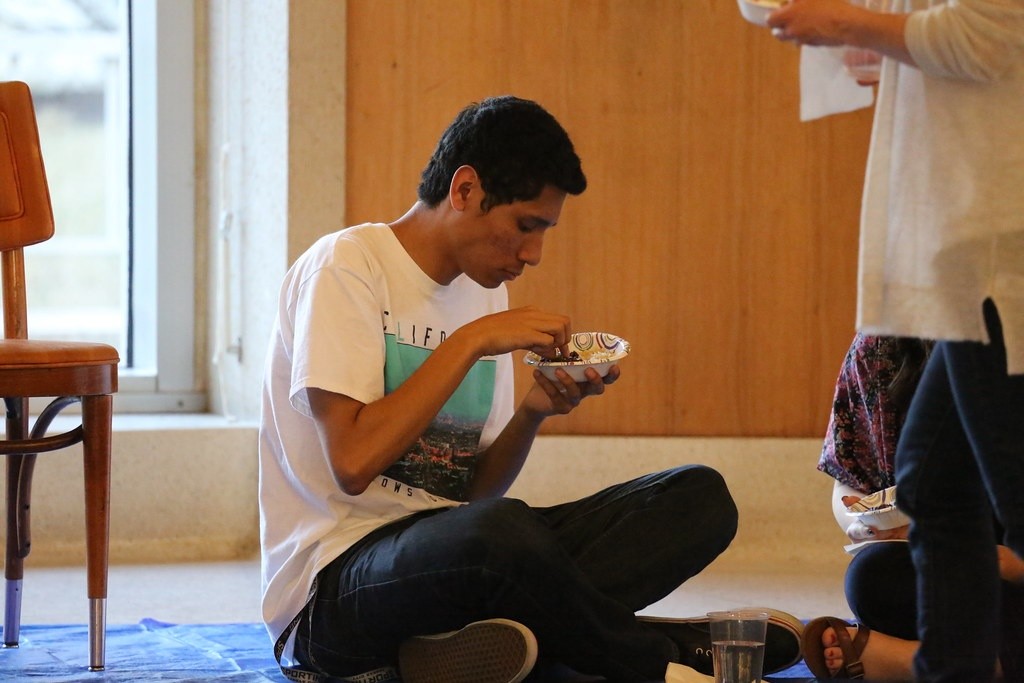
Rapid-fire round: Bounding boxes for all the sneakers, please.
[632,608,808,680]
[396,618,538,681]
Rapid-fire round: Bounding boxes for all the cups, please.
[706,611,770,683]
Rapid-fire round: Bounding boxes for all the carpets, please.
[0,616,859,683]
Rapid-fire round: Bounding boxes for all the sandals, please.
[800,615,920,683]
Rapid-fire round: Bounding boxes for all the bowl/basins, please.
[522,331,632,386]
[844,484,914,531]
[738,0,783,27]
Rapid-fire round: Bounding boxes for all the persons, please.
[257,97,807,683]
[762,0,1024,682]
[804,330,1024,682]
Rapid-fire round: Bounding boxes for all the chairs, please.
[0,80,120,672]
